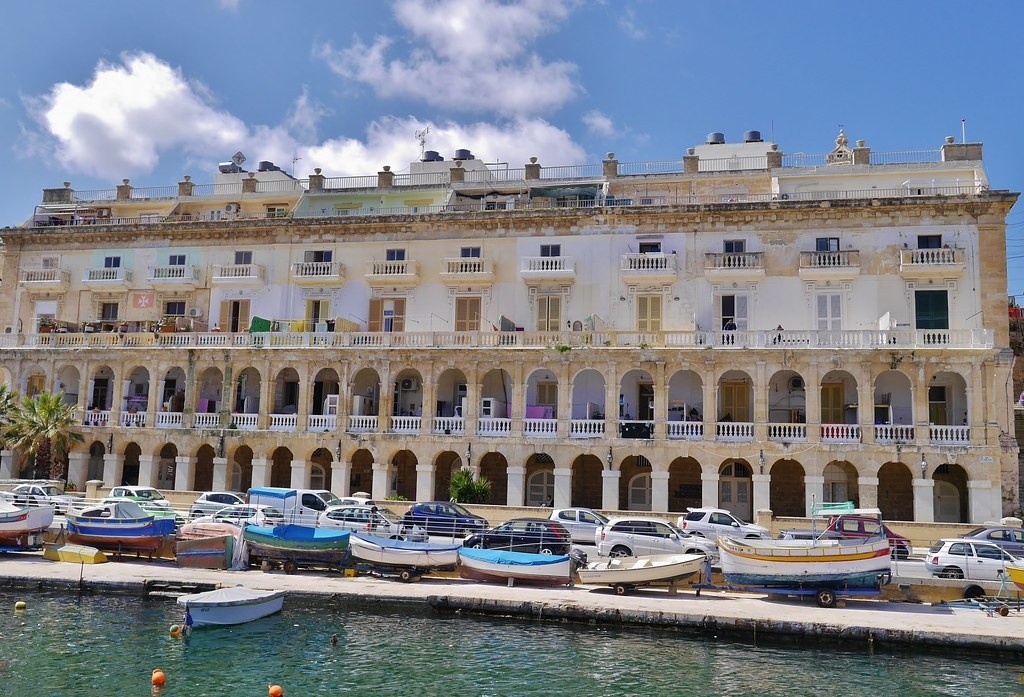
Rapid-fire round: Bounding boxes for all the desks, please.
[130,320,147,333]
[100,321,118,334]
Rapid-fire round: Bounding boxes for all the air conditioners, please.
[458,384,467,392]
[788,377,804,390]
[97,209,109,216]
[3,325,16,334]
[224,204,238,214]
[400,377,419,391]
[189,308,200,315]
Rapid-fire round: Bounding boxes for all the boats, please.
[42,541,110,564]
[243,487,350,563]
[64,498,177,554]
[349,527,461,573]
[176,583,288,625]
[0,495,56,549]
[715,491,894,590]
[457,546,573,589]
[569,549,708,584]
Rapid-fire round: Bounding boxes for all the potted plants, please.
[184,324,193,332]
[120,321,128,334]
[161,317,176,333]
[557,345,572,355]
[40,317,52,334]
[67,481,77,492]
[639,342,648,361]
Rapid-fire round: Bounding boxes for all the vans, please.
[246,486,346,528]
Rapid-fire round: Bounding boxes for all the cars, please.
[95,484,186,533]
[10,481,85,515]
[954,525,1024,560]
[462,516,572,556]
[777,528,848,540]
[925,538,1024,582]
[317,503,430,543]
[326,497,376,505]
[411,500,490,539]
[189,491,247,520]
[190,504,287,528]
[547,508,611,543]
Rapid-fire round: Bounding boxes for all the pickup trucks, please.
[826,514,913,560]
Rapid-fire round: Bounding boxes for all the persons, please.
[545,495,554,507]
[722,319,737,346]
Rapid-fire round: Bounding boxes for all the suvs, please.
[595,515,720,565]
[676,507,773,548]
